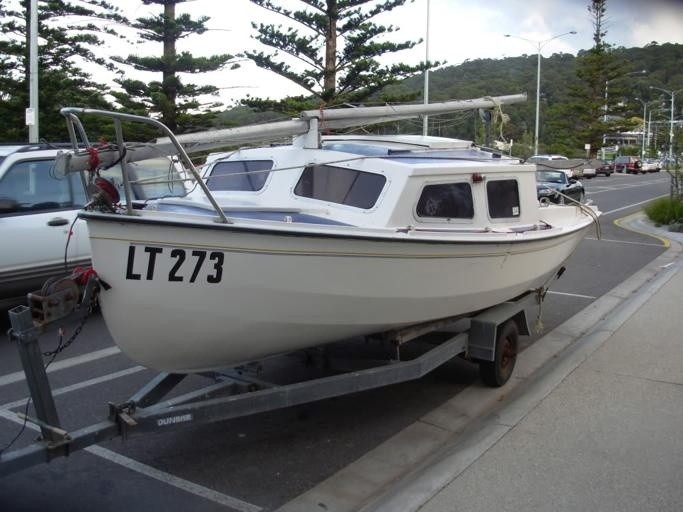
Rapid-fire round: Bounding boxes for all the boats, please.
[61,92,602,373]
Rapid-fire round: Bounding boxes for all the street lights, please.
[650,85,683,166]
[634,96,646,160]
[601,69,648,160]
[503,30,578,155]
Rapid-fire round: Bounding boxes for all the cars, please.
[0,143,195,340]
[522,154,662,204]
